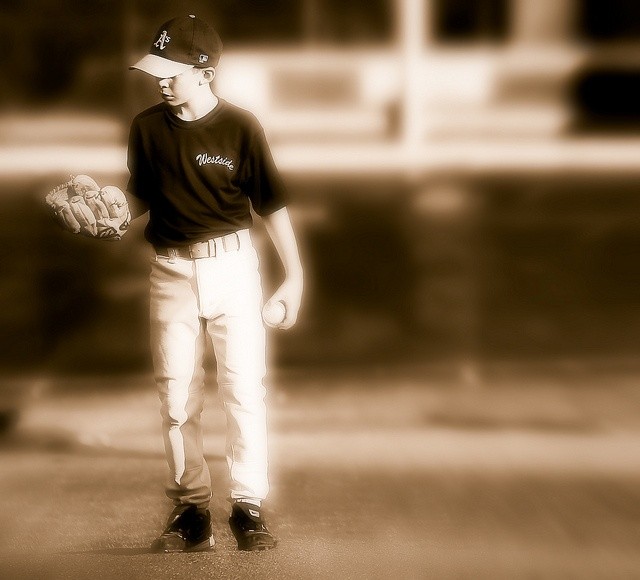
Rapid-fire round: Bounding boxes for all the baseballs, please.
[261,300,286,328]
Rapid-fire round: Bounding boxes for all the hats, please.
[128,14,225,80]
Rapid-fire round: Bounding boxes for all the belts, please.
[155,229,252,259]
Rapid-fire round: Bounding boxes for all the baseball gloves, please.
[44,174,132,241]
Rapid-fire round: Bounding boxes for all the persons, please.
[46,14,304,553]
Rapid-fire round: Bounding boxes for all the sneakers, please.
[151,505,215,555]
[229,501,277,550]
